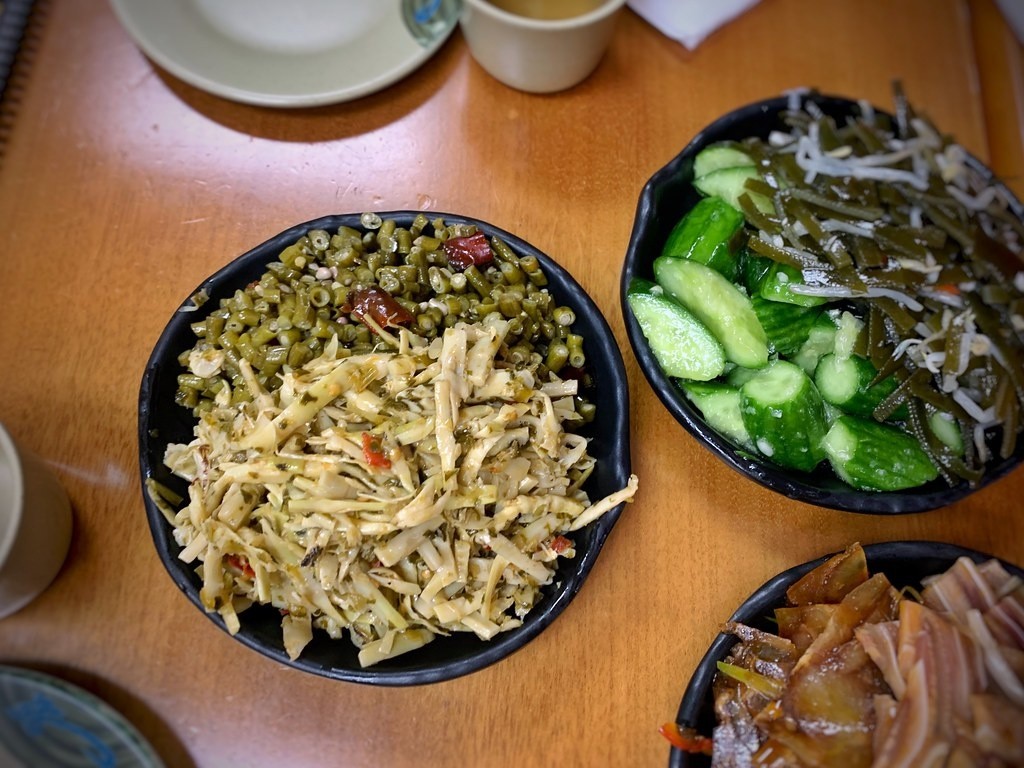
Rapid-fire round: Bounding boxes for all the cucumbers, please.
[628,140,965,491]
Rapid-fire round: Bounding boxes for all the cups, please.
[0,418,76,625]
[461,1,630,97]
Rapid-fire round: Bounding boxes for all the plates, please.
[107,1,462,112]
[1,660,171,768]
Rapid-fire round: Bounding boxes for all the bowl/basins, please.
[620,88,1024,516]
[134,209,635,688]
[668,538,1024,768]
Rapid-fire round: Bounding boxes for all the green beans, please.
[167,210,594,424]
